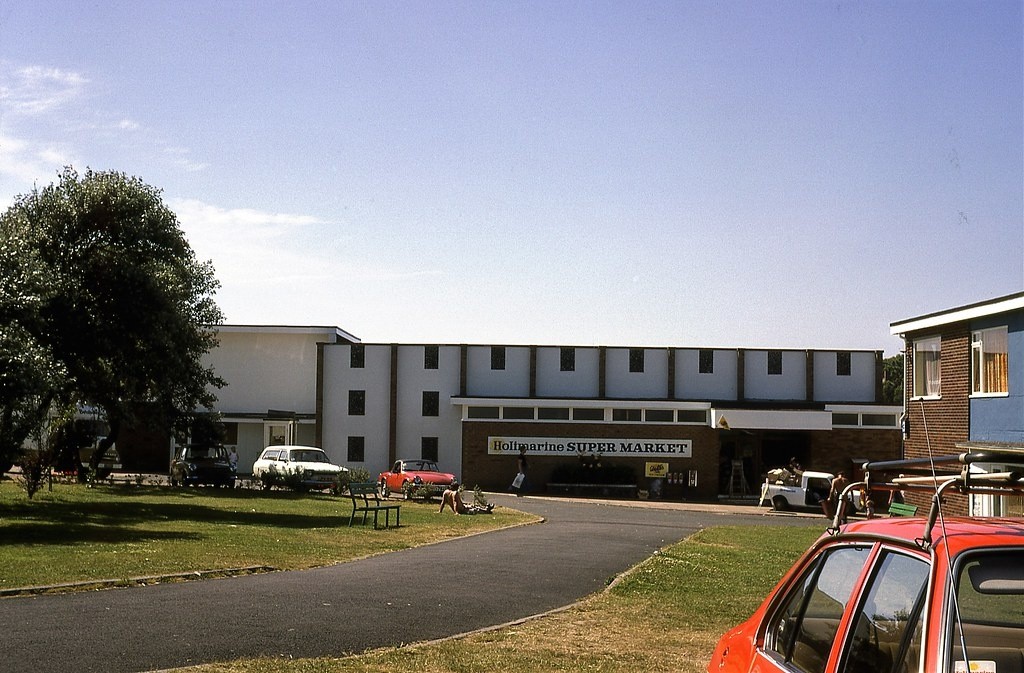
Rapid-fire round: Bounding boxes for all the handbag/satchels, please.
[512,472,525,488]
[821,501,835,521]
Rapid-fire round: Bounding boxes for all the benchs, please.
[344,481,404,529]
[793,615,1024,654]
[888,502,918,517]
[831,642,1024,673]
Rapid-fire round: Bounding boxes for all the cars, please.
[706,451,1024,673]
[170,441,237,488]
[252,445,349,497]
[376,459,458,501]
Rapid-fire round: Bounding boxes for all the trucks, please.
[0,396,122,480]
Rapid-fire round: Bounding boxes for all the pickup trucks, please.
[761,471,864,516]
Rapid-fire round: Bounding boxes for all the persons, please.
[436,482,494,516]
[228,446,239,472]
[866,496,874,519]
[517,446,530,497]
[888,490,905,517]
[791,457,808,478]
[828,472,854,525]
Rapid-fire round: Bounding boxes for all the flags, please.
[719,416,729,429]
